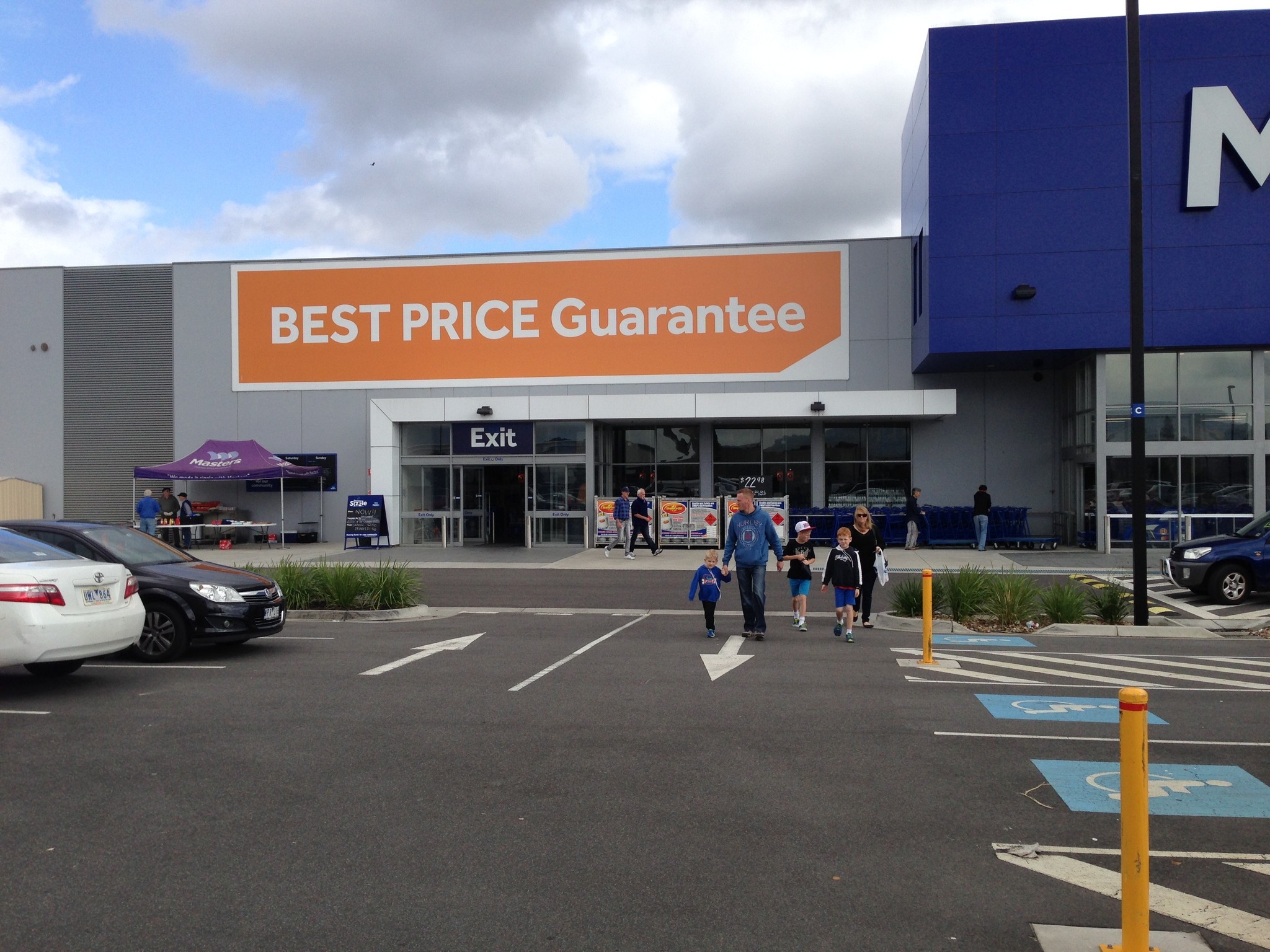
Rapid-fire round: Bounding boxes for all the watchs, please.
[779,557,784,562]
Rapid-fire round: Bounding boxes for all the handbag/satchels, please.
[873,548,889,586]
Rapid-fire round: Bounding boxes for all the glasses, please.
[858,514,867,517]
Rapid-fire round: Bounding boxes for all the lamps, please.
[811,402,825,410]
[1012,285,1036,299]
[477,406,493,416]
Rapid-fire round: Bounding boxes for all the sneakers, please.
[799,622,807,631]
[792,618,799,627]
[706,629,715,637]
[653,549,663,556]
[834,623,843,636]
[630,552,635,556]
[714,619,715,625]
[754,631,765,640]
[624,554,635,559]
[742,630,754,637]
[604,546,610,557]
[846,633,854,642]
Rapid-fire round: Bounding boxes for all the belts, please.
[622,519,625,521]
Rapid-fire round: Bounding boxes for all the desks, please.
[134,511,277,550]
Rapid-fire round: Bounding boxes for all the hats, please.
[795,521,816,533]
[162,487,172,491]
[177,492,187,497]
[621,487,630,492]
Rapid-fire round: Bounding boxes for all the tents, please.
[133,438,324,549]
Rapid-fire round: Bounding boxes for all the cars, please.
[534,482,586,512]
[828,480,907,508]
[1083,479,1270,542]
[0,518,288,678]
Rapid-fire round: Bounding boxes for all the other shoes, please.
[174,545,182,549]
[853,616,858,622]
[912,546,919,549]
[979,548,987,551]
[863,623,872,628]
[905,547,915,550]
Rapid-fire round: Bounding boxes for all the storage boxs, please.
[186,513,204,525]
[191,501,220,511]
[280,530,298,543]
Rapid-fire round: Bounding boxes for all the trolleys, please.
[788,505,1035,551]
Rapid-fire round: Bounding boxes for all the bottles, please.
[868,488,906,509]
[828,494,867,508]
[161,516,180,525]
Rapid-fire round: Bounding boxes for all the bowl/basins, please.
[254,535,268,543]
[240,521,253,524]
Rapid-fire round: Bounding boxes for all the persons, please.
[689,550,731,637]
[136,489,161,537]
[157,487,181,548]
[973,485,991,551]
[782,520,815,631]
[605,487,635,560]
[629,488,663,556]
[905,488,926,550]
[177,492,193,549]
[721,488,784,639]
[848,505,886,629]
[821,527,863,643]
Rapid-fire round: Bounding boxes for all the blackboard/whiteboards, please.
[345,495,389,538]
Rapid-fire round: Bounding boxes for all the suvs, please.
[1159,511,1270,605]
